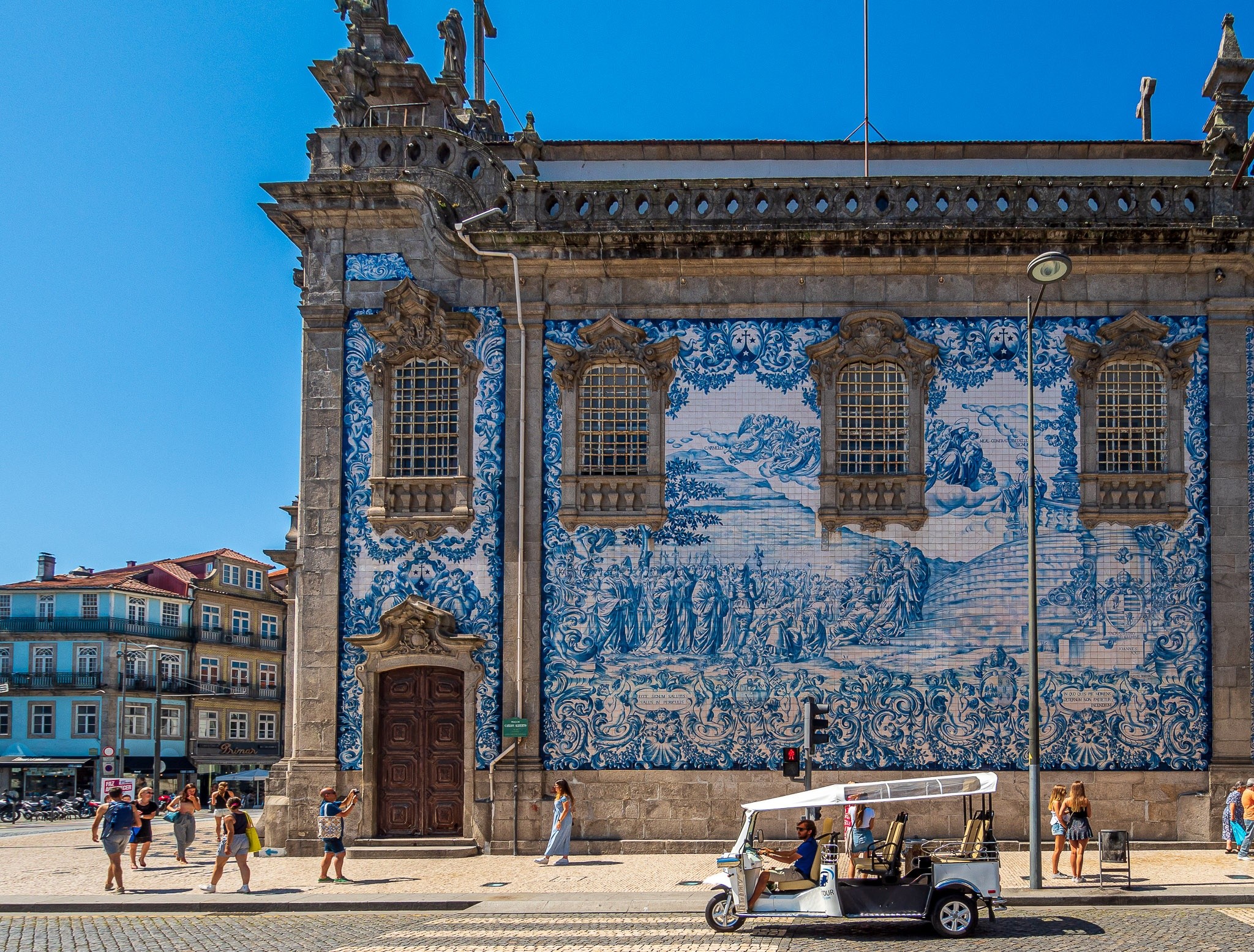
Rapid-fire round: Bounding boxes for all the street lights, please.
[117,637,162,780]
[1020,250,1079,889]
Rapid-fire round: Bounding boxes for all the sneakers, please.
[553,858,568,865]
[534,857,548,864]
[318,877,334,882]
[334,876,353,884]
[105,883,114,890]
[200,885,216,893]
[111,887,124,894]
[237,885,250,893]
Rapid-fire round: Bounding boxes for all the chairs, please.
[777,817,832,892]
[932,808,995,865]
[855,812,907,877]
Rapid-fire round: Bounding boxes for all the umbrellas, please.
[215,768,269,804]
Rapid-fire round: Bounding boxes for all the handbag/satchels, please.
[243,811,262,852]
[317,803,342,839]
[1230,820,1247,845]
[163,797,181,822]
[1064,822,1069,834]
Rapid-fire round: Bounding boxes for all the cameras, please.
[353,790,360,795]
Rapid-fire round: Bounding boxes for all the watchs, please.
[140,815,144,818]
[558,819,562,823]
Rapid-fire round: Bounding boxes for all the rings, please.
[189,796,191,798]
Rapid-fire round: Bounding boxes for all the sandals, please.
[1225,848,1240,854]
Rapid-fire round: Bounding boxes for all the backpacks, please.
[109,801,133,831]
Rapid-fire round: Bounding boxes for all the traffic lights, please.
[782,745,800,777]
[803,702,830,749]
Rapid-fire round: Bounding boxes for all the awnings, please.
[0,756,91,768]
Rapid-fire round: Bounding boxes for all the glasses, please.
[327,791,336,794]
[554,785,559,788]
[147,792,154,794]
[797,827,808,832]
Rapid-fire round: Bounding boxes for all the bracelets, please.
[226,843,231,848]
[351,803,355,806]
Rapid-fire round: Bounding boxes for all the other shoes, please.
[139,858,146,867]
[1052,871,1068,879]
[1238,856,1253,861]
[1071,876,1086,883]
[174,850,188,864]
[131,863,138,869]
[216,838,220,842]
[747,907,752,913]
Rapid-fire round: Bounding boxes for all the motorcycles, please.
[0,787,102,822]
[157,793,176,812]
[700,769,1008,939]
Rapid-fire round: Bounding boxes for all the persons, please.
[534,778,574,866]
[847,792,875,879]
[158,792,176,812]
[166,783,201,865]
[247,790,256,809]
[748,819,818,911]
[1048,780,1093,884]
[91,786,142,895]
[129,787,159,869]
[211,782,234,842]
[4,789,19,798]
[104,795,131,803]
[1222,778,1254,861]
[317,787,359,884]
[199,795,251,893]
